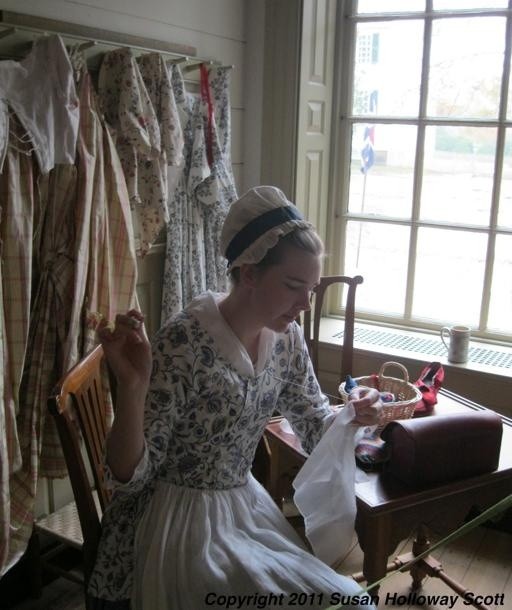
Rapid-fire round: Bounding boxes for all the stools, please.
[32,488,106,600]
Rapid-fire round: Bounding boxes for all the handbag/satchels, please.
[378,408,504,491]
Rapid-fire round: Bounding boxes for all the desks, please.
[254,385,511,605]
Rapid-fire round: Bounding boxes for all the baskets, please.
[338,359,422,429]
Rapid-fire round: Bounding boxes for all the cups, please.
[440,324,471,364]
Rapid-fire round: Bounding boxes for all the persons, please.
[94,182,383,607]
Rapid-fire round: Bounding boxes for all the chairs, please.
[49,341,129,608]
[293,275,363,406]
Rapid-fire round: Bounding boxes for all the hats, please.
[218,184,315,276]
